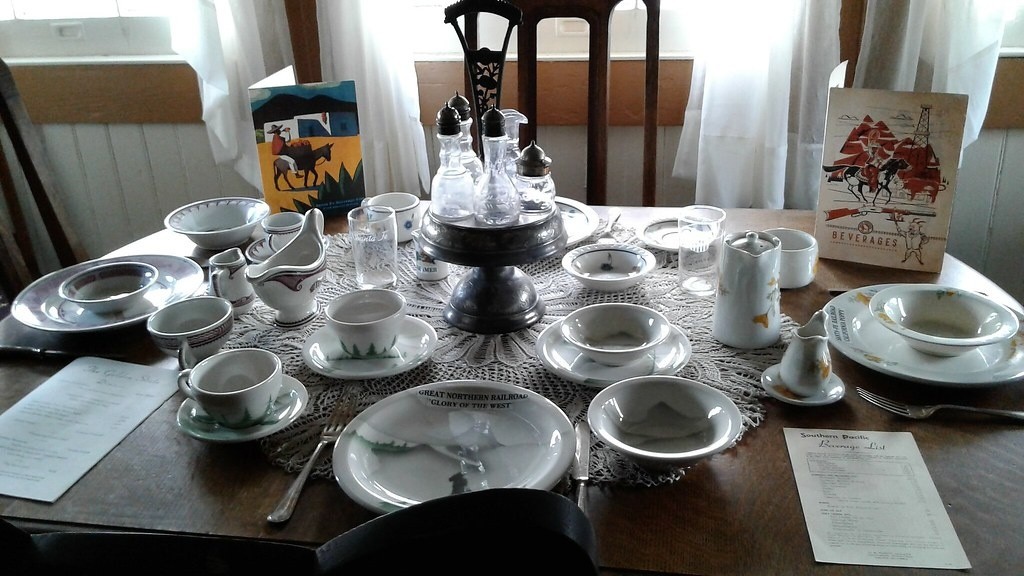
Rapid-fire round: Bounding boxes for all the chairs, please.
[0,472,600,576]
[0,56,77,315]
[442,0,661,208]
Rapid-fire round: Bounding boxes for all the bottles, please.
[430,86,555,227]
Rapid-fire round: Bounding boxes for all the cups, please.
[762,228,818,289]
[361,191,420,243]
[411,229,450,281]
[347,205,398,290]
[261,212,305,256]
[780,309,832,398]
[677,205,726,296]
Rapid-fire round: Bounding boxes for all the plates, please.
[761,363,846,406]
[554,197,600,249]
[10,255,204,333]
[244,234,329,264]
[823,285,1024,419]
[176,283,744,523]
[636,217,720,253]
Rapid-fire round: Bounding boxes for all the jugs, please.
[245,208,326,331]
[711,230,781,350]
[208,247,255,315]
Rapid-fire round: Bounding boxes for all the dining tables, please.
[0,206,1024,576]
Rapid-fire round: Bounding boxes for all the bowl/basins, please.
[147,297,233,370]
[58,262,158,314]
[164,197,271,251]
[561,244,657,292]
